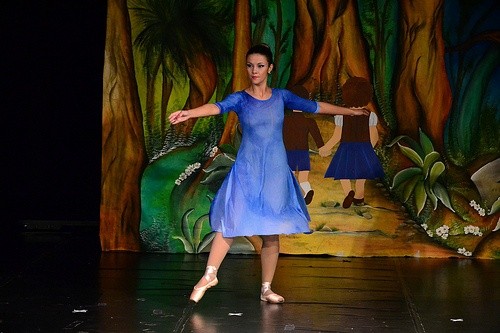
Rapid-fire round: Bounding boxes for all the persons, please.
[167,43,371,303]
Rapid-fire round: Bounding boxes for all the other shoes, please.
[260,285,285,303]
[190,275,218,303]
